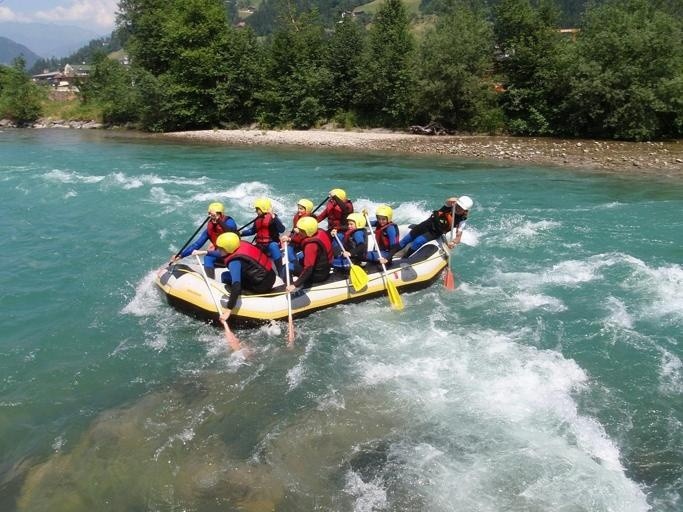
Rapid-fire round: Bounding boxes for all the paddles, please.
[333,232,369,291]
[196,254,240,350]
[364,210,404,308]
[284,240,294,348]
[443,204,456,289]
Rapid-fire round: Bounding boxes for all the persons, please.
[315,189,353,232]
[392,196,473,259]
[361,205,399,264]
[171,203,241,280]
[279,216,333,293]
[239,198,285,276]
[288,198,316,239]
[331,213,368,281]
[192,232,276,324]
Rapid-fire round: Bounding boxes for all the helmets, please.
[346,212,366,229]
[208,202,223,220]
[297,216,318,237]
[297,198,314,214]
[216,232,240,254]
[457,196,473,211]
[253,199,271,214]
[376,205,393,223]
[330,189,346,202]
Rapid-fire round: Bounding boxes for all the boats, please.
[153,241,450,329]
